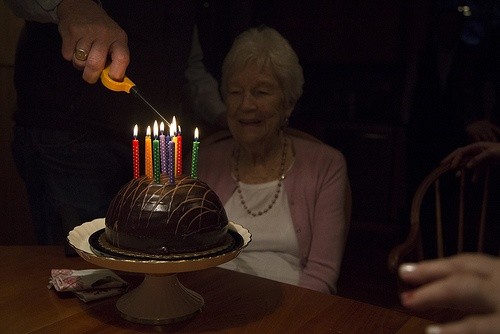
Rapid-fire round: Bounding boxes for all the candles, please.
[132,115,200,184]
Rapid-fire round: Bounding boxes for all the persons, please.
[182,27,346,296]
[5,0,229,245]
[399,254,500,334]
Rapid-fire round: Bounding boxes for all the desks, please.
[0,243,439,334]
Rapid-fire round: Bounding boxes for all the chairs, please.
[387,157,500,323]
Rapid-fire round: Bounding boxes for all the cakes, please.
[104,172,229,255]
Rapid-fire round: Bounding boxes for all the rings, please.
[74,49,87,61]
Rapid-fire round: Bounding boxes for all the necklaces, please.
[235,136,287,216]
